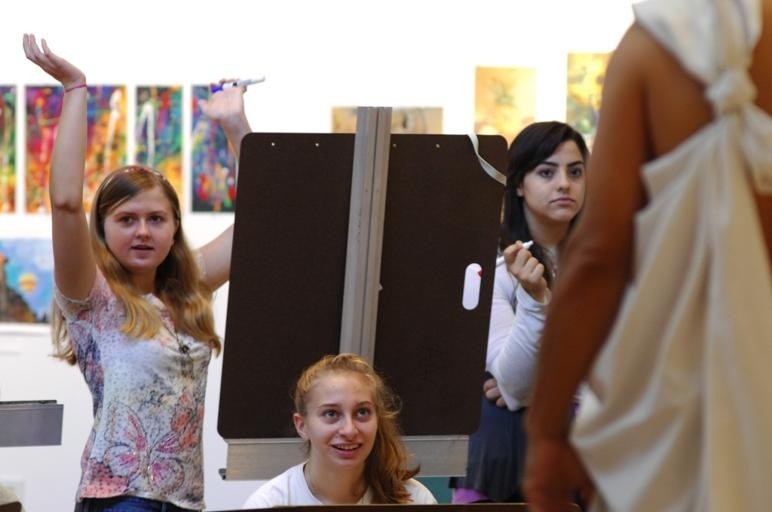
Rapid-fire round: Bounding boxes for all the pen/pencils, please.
[495,239,536,267]
[210,76,266,93]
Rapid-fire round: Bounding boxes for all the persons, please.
[21,33,255,512]
[242,352,439,511]
[465,120,591,503]
[522,1,772,512]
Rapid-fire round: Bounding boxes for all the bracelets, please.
[64,83,88,92]
